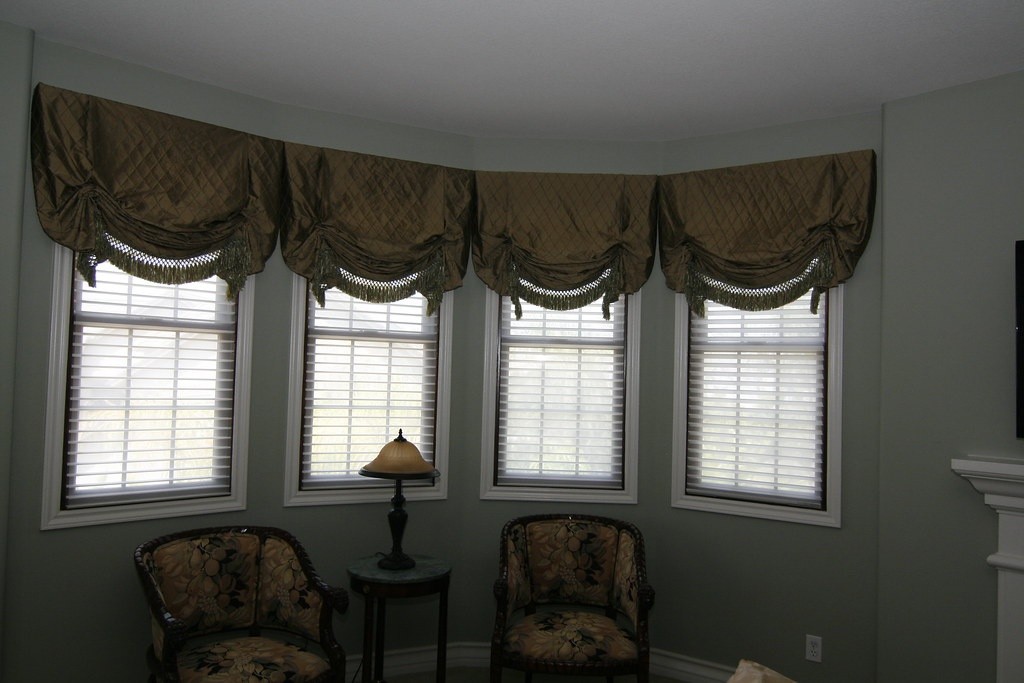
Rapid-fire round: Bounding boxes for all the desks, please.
[347,554,452,683]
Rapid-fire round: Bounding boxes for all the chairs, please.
[489,514,655,683]
[132,525,350,683]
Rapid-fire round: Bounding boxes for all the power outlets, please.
[804,634,823,663]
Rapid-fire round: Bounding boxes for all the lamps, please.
[358,428,441,569]
